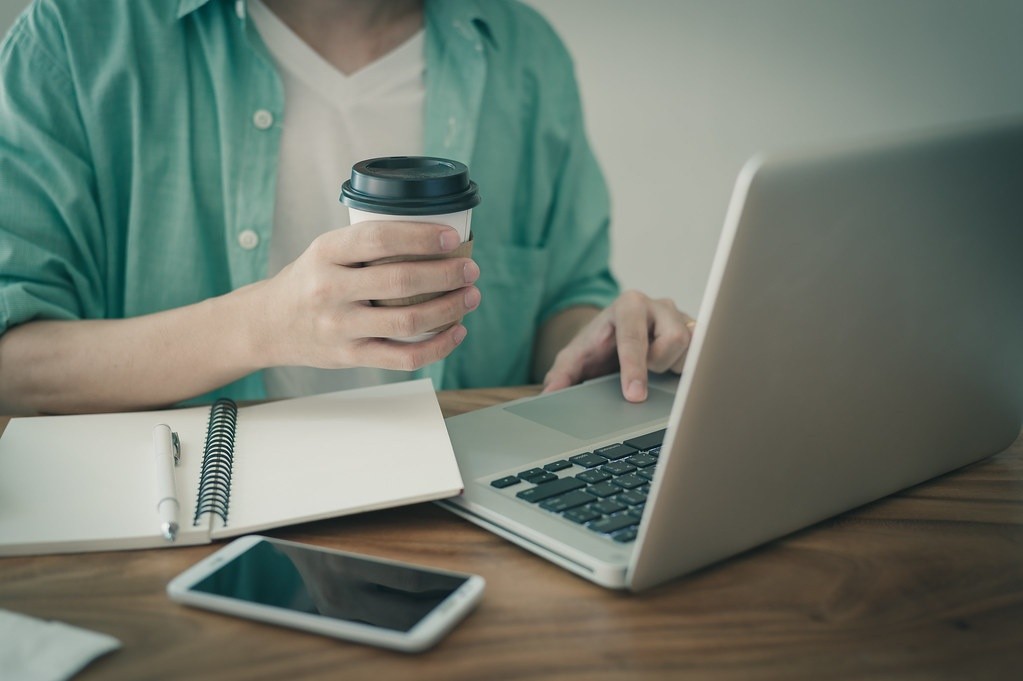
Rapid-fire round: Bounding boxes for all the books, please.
[0,378,465,557]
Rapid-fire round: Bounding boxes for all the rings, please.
[686,320,697,329]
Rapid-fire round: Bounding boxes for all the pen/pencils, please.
[150,424,182,543]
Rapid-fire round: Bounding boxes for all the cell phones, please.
[166,534,488,655]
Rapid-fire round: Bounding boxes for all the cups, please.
[339,156,483,344]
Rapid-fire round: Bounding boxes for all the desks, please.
[0,384,1022,681]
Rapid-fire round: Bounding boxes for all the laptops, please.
[427,110,1022,593]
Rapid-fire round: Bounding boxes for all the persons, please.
[1,2,701,403]
